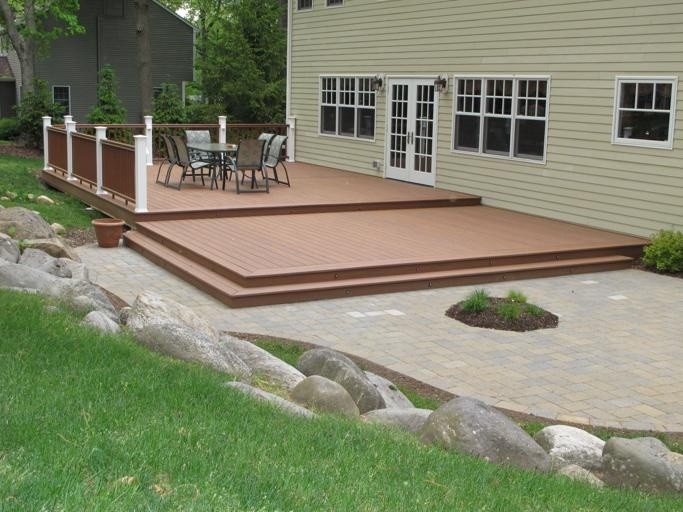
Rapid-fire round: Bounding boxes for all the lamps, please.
[371,76,385,91]
[432,77,447,92]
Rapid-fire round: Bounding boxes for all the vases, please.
[91,217,125,247]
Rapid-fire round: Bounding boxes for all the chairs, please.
[157,130,290,193]
